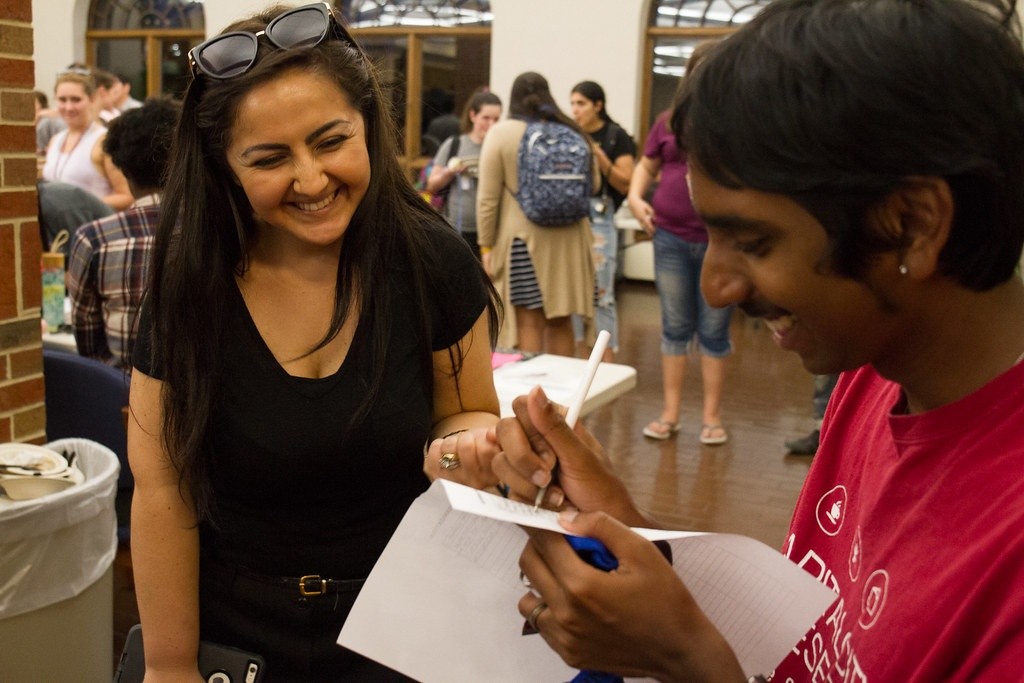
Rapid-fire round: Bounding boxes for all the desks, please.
[493,354,638,425]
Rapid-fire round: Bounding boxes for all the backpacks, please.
[503,107,592,227]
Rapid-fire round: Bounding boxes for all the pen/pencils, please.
[534,330,609,514]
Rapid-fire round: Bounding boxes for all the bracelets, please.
[439,429,468,438]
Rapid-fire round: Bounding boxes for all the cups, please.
[41,229,70,334]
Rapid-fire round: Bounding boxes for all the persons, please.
[126,3,500,683]
[428,94,461,159]
[487,0,1024,683]
[30,61,186,382]
[428,72,638,367]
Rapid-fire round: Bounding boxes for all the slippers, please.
[642,419,681,440]
[699,424,727,443]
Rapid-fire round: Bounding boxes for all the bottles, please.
[461,168,471,189]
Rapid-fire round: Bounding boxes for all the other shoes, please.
[784,430,821,453]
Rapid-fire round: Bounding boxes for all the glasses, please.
[57,68,90,78]
[187,3,367,96]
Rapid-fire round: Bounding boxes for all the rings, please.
[439,453,460,471]
[528,602,548,631]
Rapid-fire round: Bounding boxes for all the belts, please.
[208,548,369,596]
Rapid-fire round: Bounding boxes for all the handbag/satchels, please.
[412,135,460,213]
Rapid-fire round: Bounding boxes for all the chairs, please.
[43,349,133,549]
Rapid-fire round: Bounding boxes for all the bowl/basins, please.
[0,442,83,501]
